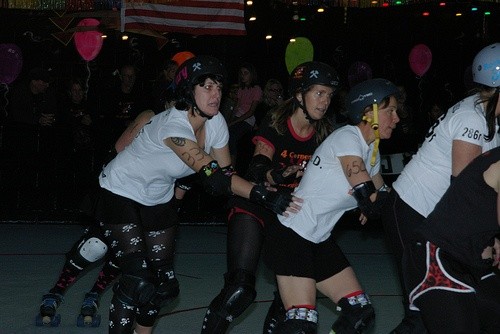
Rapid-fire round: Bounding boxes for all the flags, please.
[120,0,248,37]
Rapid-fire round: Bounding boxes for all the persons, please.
[33,43,500,334]
[401,146,500,334]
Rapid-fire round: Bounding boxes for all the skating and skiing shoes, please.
[35,291,61,327]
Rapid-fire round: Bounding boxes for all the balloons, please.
[284,37,315,76]
[172,51,195,67]
[0,42,23,84]
[408,45,433,77]
[72,18,104,63]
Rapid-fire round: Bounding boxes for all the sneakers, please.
[77,290,101,327]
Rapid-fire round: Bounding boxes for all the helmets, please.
[288,62,339,94]
[174,57,224,100]
[471,43,500,87]
[172,51,195,67]
[345,79,401,125]
